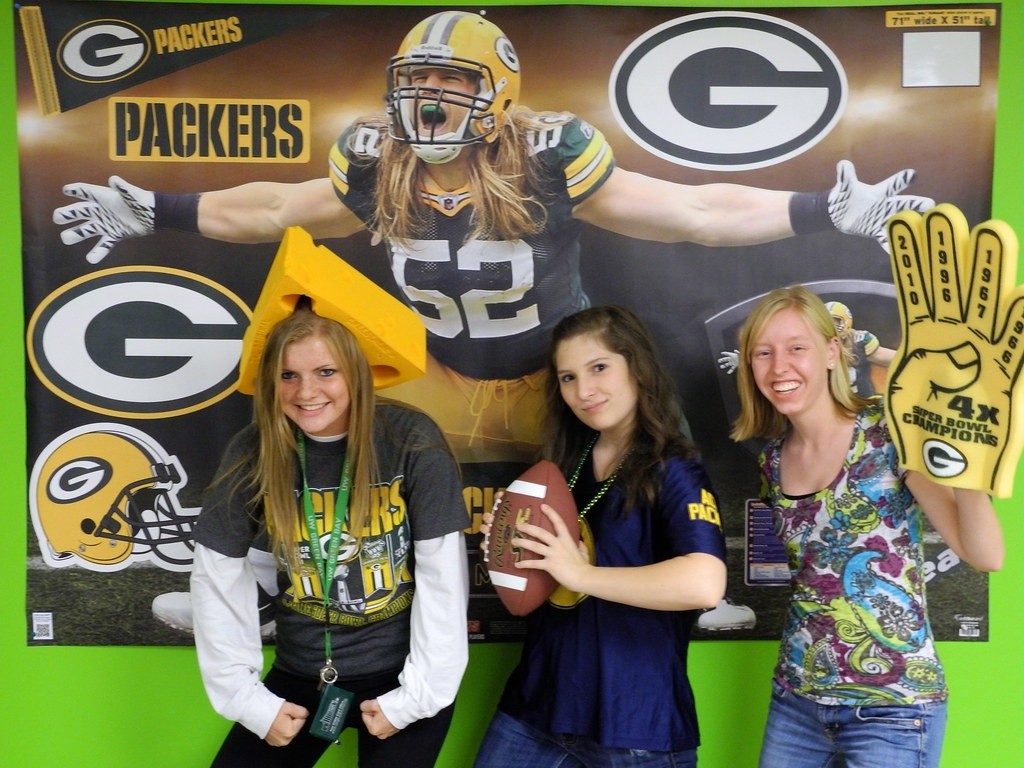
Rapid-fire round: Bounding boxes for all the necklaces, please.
[567,432,637,523]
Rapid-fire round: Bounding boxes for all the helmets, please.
[382,11,524,162]
[824,301,852,336]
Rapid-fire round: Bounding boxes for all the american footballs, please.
[482,459,581,617]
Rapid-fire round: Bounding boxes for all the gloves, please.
[51,175,199,265]
[717,349,740,376]
[883,203,1024,499]
[789,159,935,256]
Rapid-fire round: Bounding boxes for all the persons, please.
[473,309,728,768]
[52,10,937,636]
[727,289,1006,768]
[188,310,470,768]
[717,300,899,398]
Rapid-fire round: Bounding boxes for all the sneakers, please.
[151,592,278,643]
[697,600,756,629]
